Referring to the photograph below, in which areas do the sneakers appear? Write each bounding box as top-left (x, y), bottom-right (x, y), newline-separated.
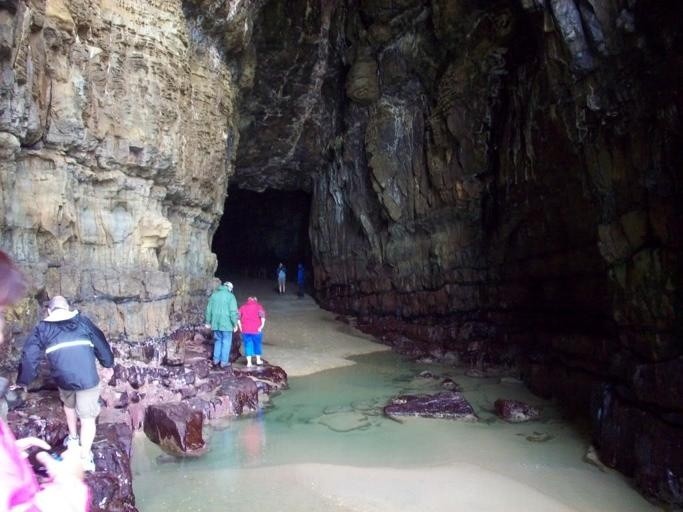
top-left (65, 437), bottom-right (78, 454)
top-left (247, 364), bottom-right (251, 368)
top-left (220, 361), bottom-right (231, 368)
top-left (80, 454), bottom-right (94, 472)
top-left (212, 360), bottom-right (219, 366)
top-left (257, 361), bottom-right (263, 365)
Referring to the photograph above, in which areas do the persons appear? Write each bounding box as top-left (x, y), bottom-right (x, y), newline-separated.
top-left (236, 295), bottom-right (266, 369)
top-left (7, 294), bottom-right (114, 456)
top-left (295, 262), bottom-right (305, 298)
top-left (275, 261), bottom-right (288, 297)
top-left (0, 251), bottom-right (96, 511)
top-left (238, 263), bottom-right (267, 281)
top-left (203, 281), bottom-right (238, 371)
